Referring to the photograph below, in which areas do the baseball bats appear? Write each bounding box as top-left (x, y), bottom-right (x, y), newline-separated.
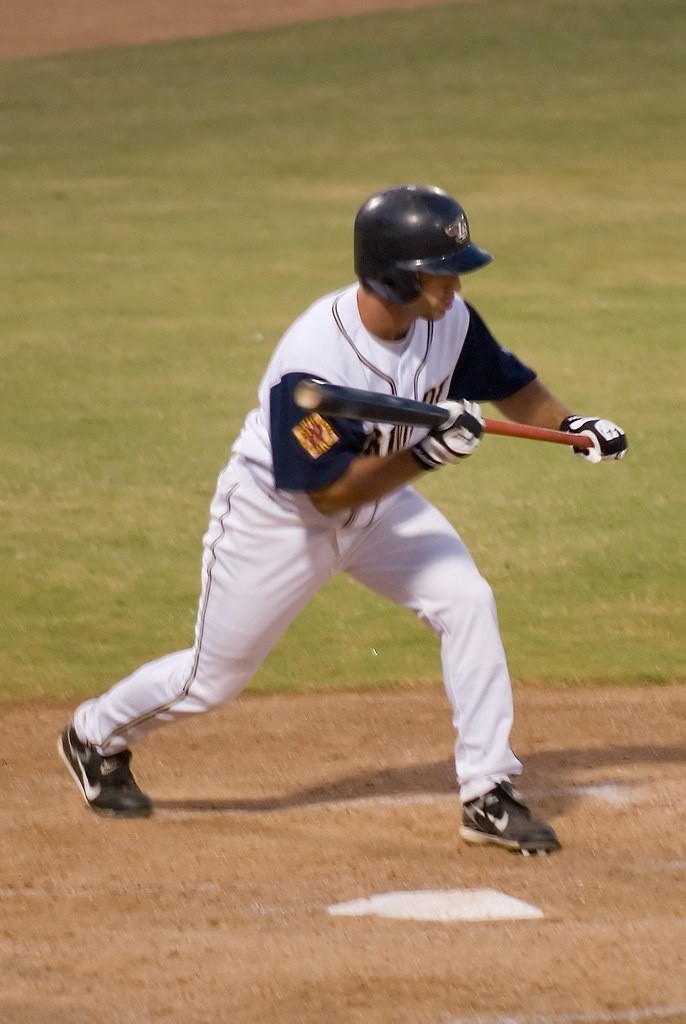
top-left (293, 375), bottom-right (593, 450)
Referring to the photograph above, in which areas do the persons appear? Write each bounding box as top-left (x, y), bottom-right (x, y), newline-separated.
top-left (58, 184), bottom-right (632, 854)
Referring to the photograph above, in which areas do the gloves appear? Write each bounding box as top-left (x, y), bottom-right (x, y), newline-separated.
top-left (559, 415), bottom-right (628, 463)
top-left (413, 398), bottom-right (488, 472)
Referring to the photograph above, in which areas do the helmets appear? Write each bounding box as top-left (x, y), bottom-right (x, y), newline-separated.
top-left (353, 186), bottom-right (491, 304)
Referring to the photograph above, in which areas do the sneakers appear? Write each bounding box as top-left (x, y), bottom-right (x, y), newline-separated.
top-left (461, 781), bottom-right (560, 856)
top-left (56, 720), bottom-right (154, 817)
